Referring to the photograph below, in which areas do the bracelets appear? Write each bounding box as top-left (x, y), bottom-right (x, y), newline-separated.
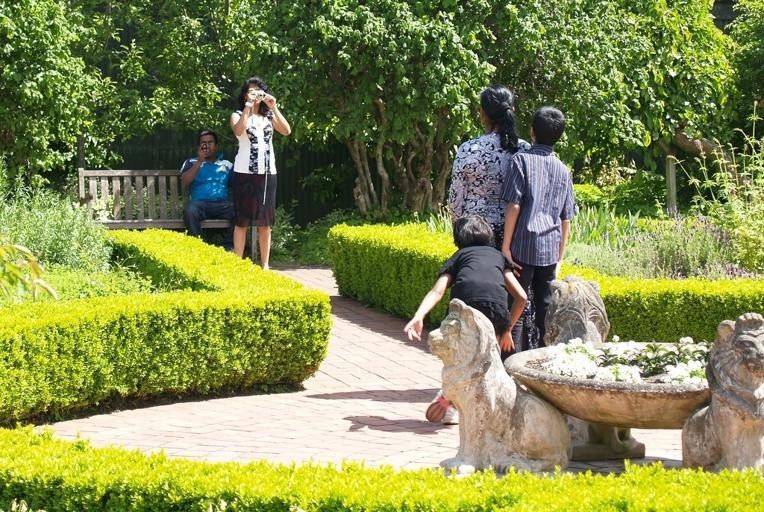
top-left (244, 100), bottom-right (254, 107)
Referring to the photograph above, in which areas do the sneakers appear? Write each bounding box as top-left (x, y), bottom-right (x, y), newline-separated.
top-left (426, 390), bottom-right (459, 425)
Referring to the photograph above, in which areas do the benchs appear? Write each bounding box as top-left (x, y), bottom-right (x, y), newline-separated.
top-left (76, 167), bottom-right (258, 265)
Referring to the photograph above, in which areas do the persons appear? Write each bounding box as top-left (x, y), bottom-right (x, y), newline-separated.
top-left (177, 129), bottom-right (239, 254)
top-left (448, 80), bottom-right (534, 262)
top-left (229, 76), bottom-right (291, 270)
top-left (502, 105), bottom-right (576, 356)
top-left (402, 212), bottom-right (529, 426)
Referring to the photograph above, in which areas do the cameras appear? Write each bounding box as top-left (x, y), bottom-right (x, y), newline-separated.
top-left (253, 89), bottom-right (267, 101)
